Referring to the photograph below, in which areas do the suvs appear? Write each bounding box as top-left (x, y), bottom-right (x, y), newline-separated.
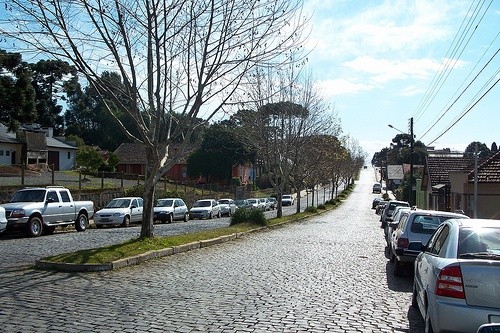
top-left (388, 210), bottom-right (471, 277)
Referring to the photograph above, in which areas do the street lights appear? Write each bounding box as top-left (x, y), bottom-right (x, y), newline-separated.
top-left (387, 124), bottom-right (414, 207)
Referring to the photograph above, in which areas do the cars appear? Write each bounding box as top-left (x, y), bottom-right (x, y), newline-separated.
top-left (407, 218), bottom-right (500, 333)
top-left (282, 195), bottom-right (294, 206)
top-left (234, 198), bottom-right (279, 212)
top-left (153, 198), bottom-right (190, 224)
top-left (217, 199), bottom-right (238, 217)
top-left (189, 199), bottom-right (222, 220)
top-left (93, 197), bottom-right (144, 229)
top-left (372, 197), bottom-right (441, 256)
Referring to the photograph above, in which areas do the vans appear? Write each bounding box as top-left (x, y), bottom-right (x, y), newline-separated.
top-left (373, 183), bottom-right (381, 193)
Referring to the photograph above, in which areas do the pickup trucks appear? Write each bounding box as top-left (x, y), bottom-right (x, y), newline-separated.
top-left (0, 186), bottom-right (95, 238)
